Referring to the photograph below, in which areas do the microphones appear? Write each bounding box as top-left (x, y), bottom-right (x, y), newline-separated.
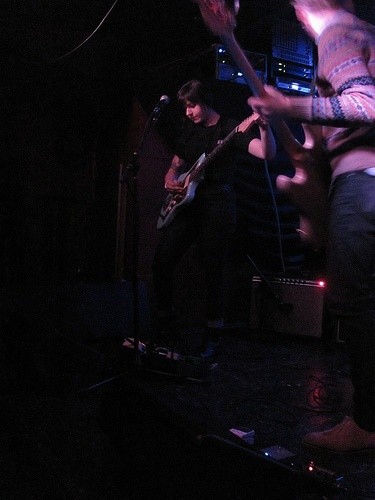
top-left (152, 95), bottom-right (170, 120)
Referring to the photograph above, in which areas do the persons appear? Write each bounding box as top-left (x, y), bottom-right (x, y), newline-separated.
top-left (247, 0), bottom-right (375, 447)
top-left (143, 80), bottom-right (276, 351)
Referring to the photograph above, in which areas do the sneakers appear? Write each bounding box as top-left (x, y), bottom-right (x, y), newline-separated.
top-left (307, 367), bottom-right (329, 411)
top-left (304, 416), bottom-right (375, 452)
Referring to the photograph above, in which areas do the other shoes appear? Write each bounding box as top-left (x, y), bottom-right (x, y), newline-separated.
top-left (207, 330), bottom-right (222, 356)
top-left (149, 332), bottom-right (162, 348)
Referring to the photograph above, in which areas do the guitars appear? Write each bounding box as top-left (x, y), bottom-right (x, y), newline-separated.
top-left (155, 112), bottom-right (258, 233)
top-left (197, 0), bottom-right (331, 252)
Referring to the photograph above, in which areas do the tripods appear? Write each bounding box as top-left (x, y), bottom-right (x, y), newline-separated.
top-left (76, 115), bottom-right (212, 395)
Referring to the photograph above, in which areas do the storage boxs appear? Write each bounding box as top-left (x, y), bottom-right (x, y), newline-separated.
top-left (249, 276), bottom-right (324, 338)
